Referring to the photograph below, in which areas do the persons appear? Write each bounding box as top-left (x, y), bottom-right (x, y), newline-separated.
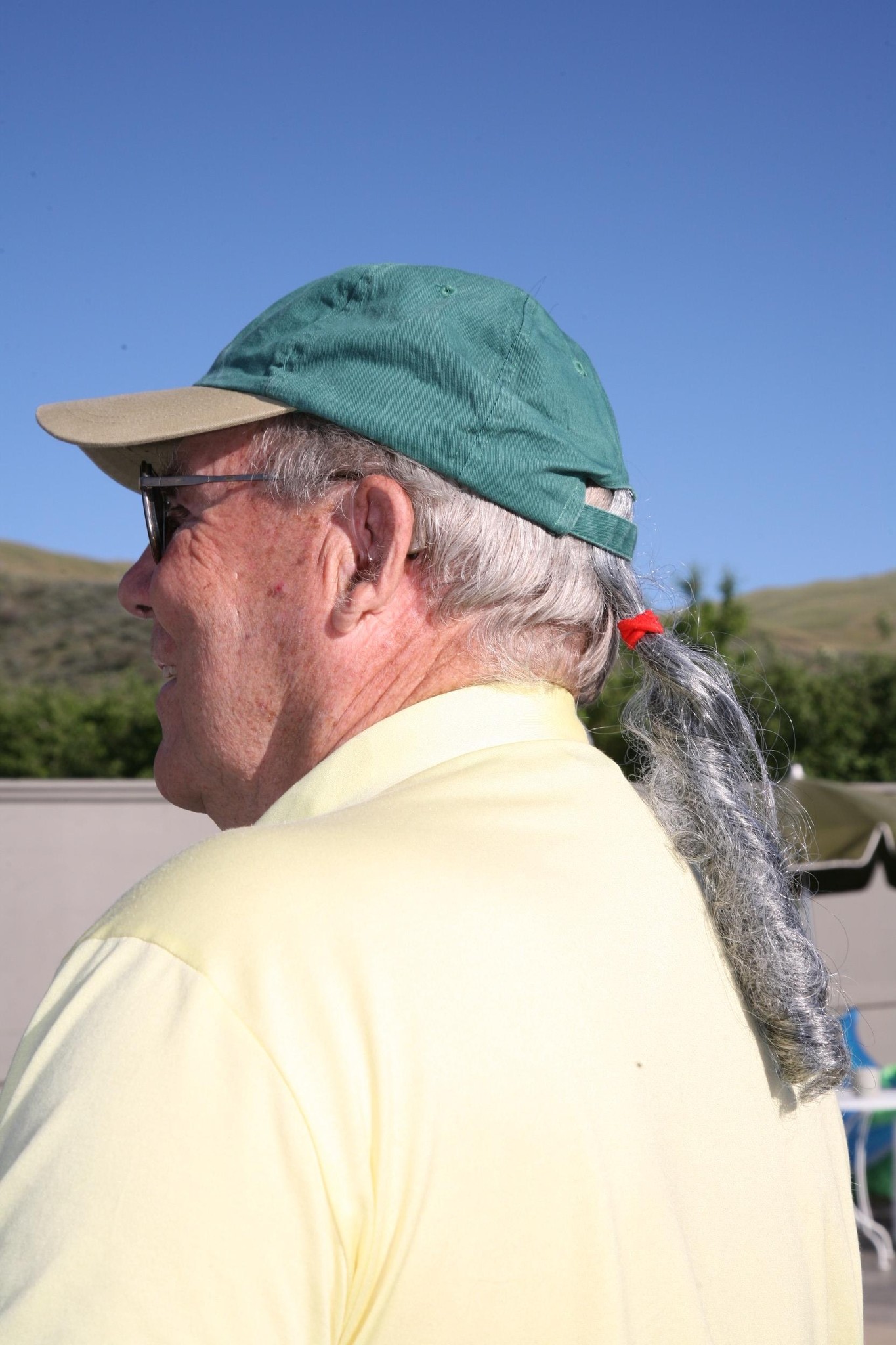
top-left (0, 268), bottom-right (869, 1344)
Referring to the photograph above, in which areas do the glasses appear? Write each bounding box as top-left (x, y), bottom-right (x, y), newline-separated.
top-left (136, 461), bottom-right (379, 552)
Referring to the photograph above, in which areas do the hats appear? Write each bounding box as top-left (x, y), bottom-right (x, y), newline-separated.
top-left (41, 266), bottom-right (646, 559)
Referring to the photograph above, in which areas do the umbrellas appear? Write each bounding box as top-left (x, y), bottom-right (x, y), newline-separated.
top-left (755, 758), bottom-right (896, 939)
